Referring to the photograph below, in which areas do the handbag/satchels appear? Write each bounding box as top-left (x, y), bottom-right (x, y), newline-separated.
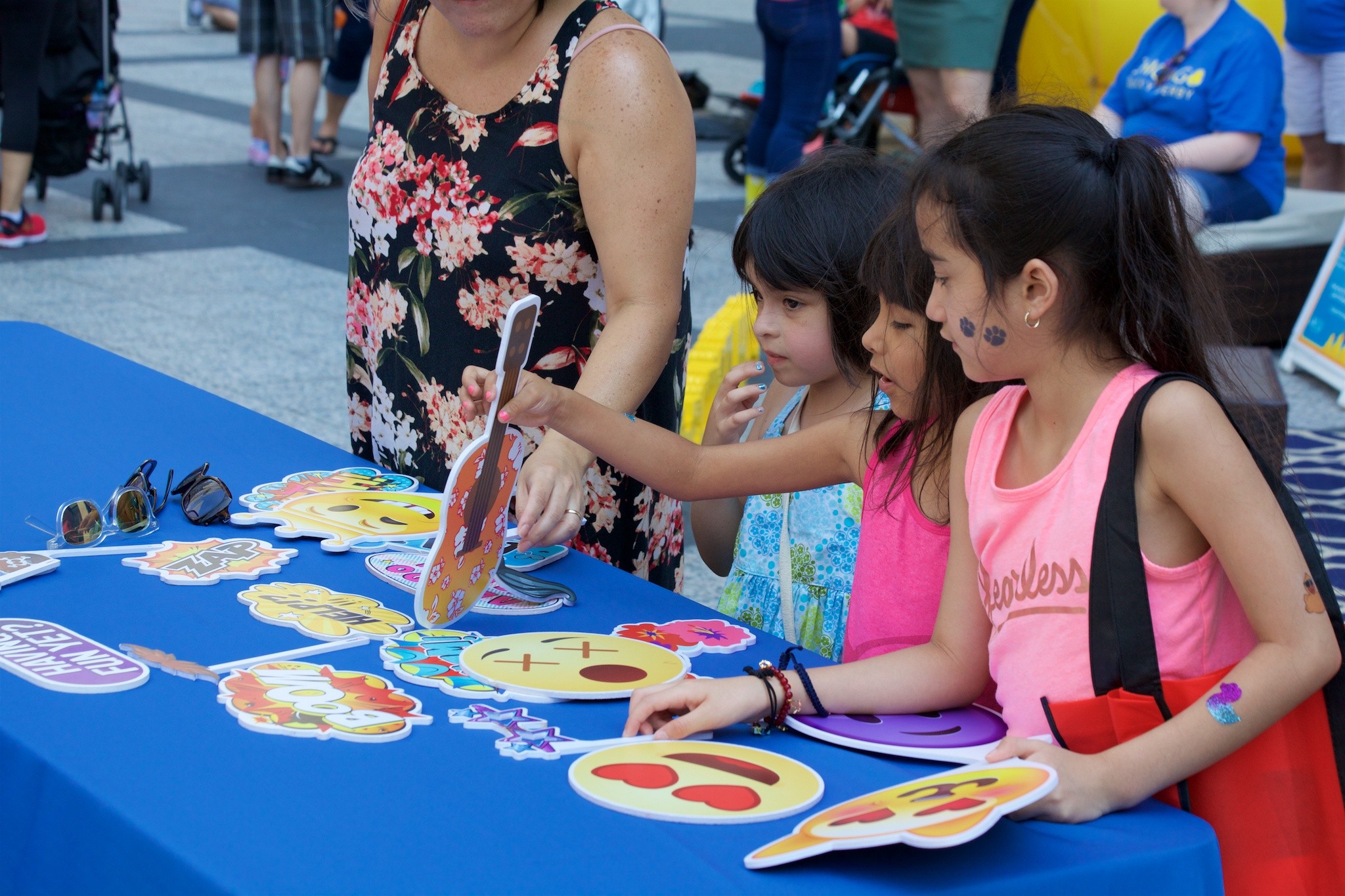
top-left (1039, 375), bottom-right (1345, 896)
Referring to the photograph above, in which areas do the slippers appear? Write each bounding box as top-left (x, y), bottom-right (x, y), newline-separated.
top-left (311, 135), bottom-right (339, 155)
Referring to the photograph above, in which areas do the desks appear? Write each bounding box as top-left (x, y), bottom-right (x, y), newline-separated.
top-left (0, 321), bottom-right (1225, 896)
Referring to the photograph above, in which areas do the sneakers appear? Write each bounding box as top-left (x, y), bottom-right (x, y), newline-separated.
top-left (1, 205), bottom-right (47, 247)
top-left (266, 139), bottom-right (290, 183)
top-left (282, 156), bottom-right (344, 188)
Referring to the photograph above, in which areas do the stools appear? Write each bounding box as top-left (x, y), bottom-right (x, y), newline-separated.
top-left (1194, 189), bottom-right (1345, 346)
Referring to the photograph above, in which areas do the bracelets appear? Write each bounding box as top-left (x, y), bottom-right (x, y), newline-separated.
top-left (740, 645), bottom-right (831, 737)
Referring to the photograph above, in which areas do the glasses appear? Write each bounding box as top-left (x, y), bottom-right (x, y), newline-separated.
top-left (25, 485), bottom-right (160, 550)
top-left (124, 459), bottom-right (174, 518)
top-left (171, 463), bottom-right (233, 526)
top-left (1158, 52), bottom-right (1186, 86)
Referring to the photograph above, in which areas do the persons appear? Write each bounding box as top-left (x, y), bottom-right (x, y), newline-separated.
top-left (183, 0), bottom-right (373, 190)
top-left (686, 147), bottom-right (917, 659)
top-left (350, 0), bottom-right (686, 588)
top-left (459, 185), bottom-right (992, 711)
top-left (725, 0), bottom-right (1345, 243)
top-left (619, 102), bottom-right (1334, 895)
top-left (1, 2), bottom-right (47, 251)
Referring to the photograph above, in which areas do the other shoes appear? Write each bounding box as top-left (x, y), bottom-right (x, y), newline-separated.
top-left (248, 140), bottom-right (269, 169)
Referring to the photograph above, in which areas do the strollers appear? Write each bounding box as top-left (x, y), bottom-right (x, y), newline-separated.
top-left (718, 0), bottom-right (910, 188)
top-left (21, 0), bottom-right (153, 222)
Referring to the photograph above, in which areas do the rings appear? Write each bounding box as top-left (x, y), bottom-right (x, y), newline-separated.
top-left (563, 508), bottom-right (583, 520)
